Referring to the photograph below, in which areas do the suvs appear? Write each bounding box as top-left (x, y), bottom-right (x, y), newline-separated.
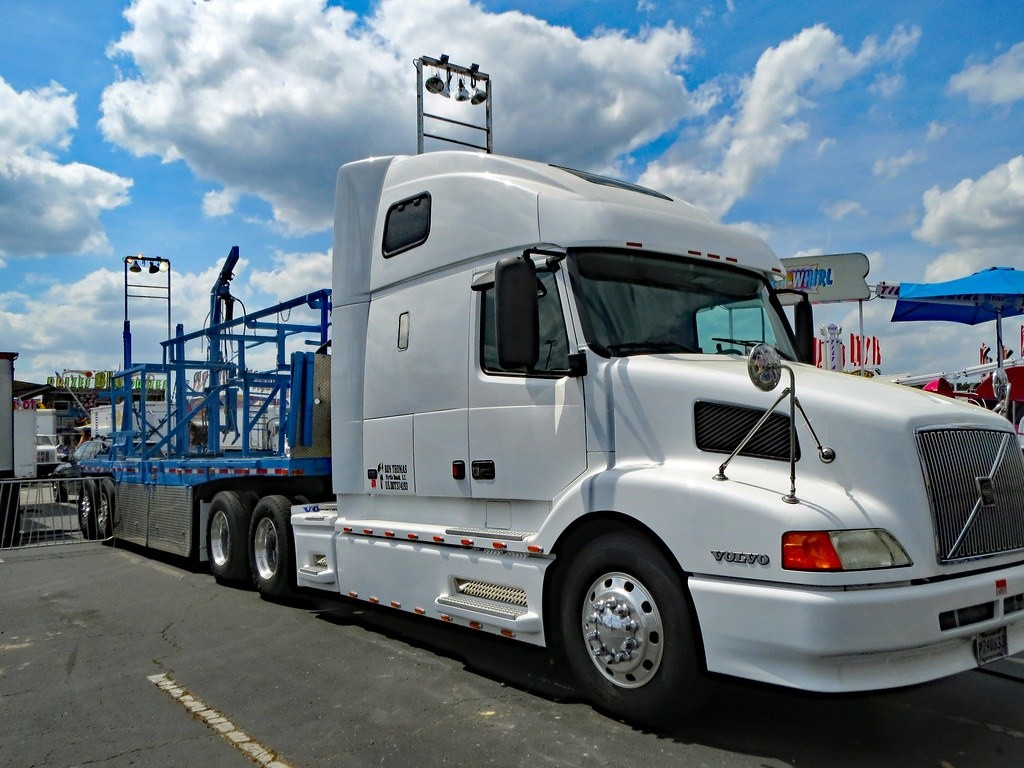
top-left (49, 440), bottom-right (169, 503)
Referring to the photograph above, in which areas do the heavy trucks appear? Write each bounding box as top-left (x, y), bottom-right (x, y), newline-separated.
top-left (72, 152), bottom-right (1023, 727)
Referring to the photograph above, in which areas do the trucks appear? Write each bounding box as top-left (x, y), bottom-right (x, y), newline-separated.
top-left (89, 400), bottom-right (178, 452)
top-left (35, 408), bottom-right (64, 466)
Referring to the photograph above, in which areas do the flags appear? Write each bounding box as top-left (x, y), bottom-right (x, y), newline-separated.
top-left (1020, 325), bottom-right (1024, 357)
top-left (813, 333), bottom-right (881, 368)
top-left (1003, 345), bottom-right (1014, 359)
top-left (980, 343), bottom-right (993, 365)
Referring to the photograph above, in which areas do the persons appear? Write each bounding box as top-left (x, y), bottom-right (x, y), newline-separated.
top-left (77, 428), bottom-right (92, 447)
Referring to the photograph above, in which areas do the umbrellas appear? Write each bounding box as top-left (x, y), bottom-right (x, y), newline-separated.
top-left (891, 266), bottom-right (1024, 367)
